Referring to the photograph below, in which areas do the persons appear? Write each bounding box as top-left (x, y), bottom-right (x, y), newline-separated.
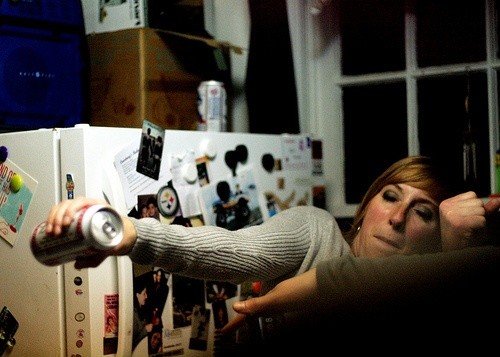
top-left (213, 191), bottom-right (500, 356)
top-left (106, 181), bottom-right (252, 357)
top-left (45, 156), bottom-right (447, 298)
top-left (139, 125), bottom-right (163, 173)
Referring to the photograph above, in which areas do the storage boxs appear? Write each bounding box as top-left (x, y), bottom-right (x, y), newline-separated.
top-left (0, 0), bottom-right (248, 130)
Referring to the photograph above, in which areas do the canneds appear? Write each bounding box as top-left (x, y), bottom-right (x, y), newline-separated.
top-left (30, 205), bottom-right (125, 266)
top-left (190, 81), bottom-right (228, 133)
